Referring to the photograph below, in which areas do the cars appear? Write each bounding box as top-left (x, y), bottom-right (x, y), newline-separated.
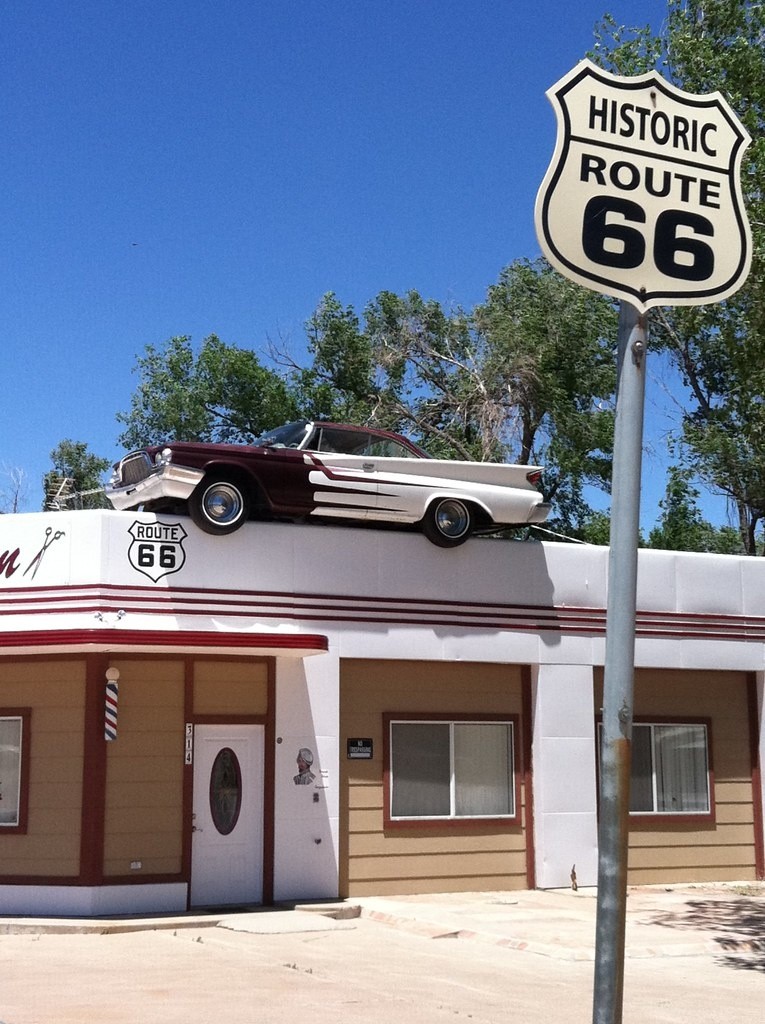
top-left (103, 420), bottom-right (559, 548)
